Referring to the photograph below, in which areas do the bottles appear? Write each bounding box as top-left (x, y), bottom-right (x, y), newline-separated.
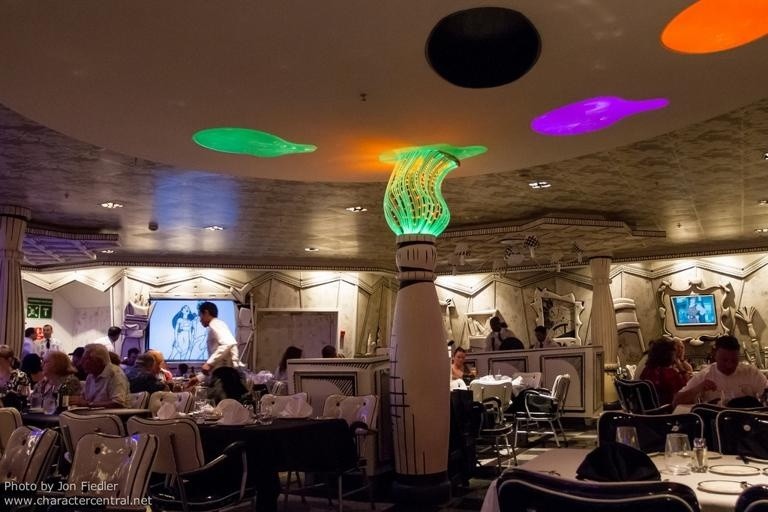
top-left (14, 383), bottom-right (81, 417)
top-left (693, 437), bottom-right (709, 474)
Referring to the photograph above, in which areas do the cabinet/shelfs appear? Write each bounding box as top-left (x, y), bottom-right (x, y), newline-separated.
top-left (466, 342), bottom-right (604, 430)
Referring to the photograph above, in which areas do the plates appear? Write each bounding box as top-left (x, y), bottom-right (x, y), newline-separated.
top-left (697, 479), bottom-right (752, 495)
top-left (646, 450), bottom-right (661, 459)
top-left (309, 415), bottom-right (335, 420)
top-left (762, 467), bottom-right (768, 476)
top-left (685, 449), bottom-right (721, 459)
top-left (707, 464), bottom-right (759, 476)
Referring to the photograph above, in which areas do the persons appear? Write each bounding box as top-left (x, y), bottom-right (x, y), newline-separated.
top-left (688, 299), bottom-right (706, 324)
top-left (0, 302), bottom-right (384, 427)
top-left (448, 313), bottom-right (768, 421)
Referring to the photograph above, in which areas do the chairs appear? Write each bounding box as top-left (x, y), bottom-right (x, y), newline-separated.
top-left (0, 357), bottom-right (768, 512)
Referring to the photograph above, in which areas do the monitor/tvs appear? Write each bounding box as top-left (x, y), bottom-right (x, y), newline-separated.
top-left (671, 293), bottom-right (718, 326)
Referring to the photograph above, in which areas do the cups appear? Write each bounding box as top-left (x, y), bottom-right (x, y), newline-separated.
top-left (495, 374), bottom-right (502, 381)
top-left (189, 387), bottom-right (275, 426)
top-left (663, 432), bottom-right (693, 475)
top-left (170, 369), bottom-right (201, 382)
top-left (613, 426), bottom-right (638, 451)
